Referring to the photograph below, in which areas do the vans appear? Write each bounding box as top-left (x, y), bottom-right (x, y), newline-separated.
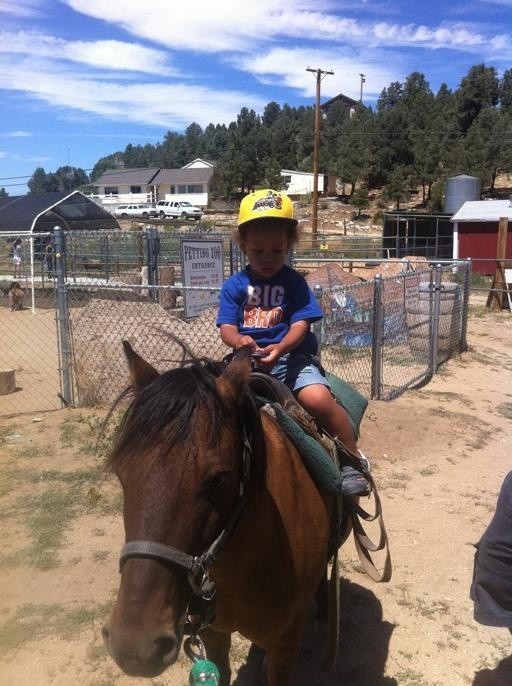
top-left (114, 204), bottom-right (157, 220)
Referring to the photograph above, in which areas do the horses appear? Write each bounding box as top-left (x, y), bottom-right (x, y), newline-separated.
top-left (79, 255), bottom-right (103, 272)
top-left (99, 328), bottom-right (360, 686)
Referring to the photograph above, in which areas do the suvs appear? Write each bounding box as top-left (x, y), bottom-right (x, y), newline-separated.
top-left (156, 200), bottom-right (202, 220)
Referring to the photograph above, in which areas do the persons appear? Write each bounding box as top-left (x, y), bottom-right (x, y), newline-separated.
top-left (13, 238), bottom-right (23, 279)
top-left (43, 237), bottom-right (55, 278)
top-left (216, 188), bottom-right (373, 497)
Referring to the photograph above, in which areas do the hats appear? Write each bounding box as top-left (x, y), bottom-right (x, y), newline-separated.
top-left (238, 189), bottom-right (299, 231)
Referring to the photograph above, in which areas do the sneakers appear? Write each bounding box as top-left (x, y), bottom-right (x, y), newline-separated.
top-left (341, 458), bottom-right (371, 496)
